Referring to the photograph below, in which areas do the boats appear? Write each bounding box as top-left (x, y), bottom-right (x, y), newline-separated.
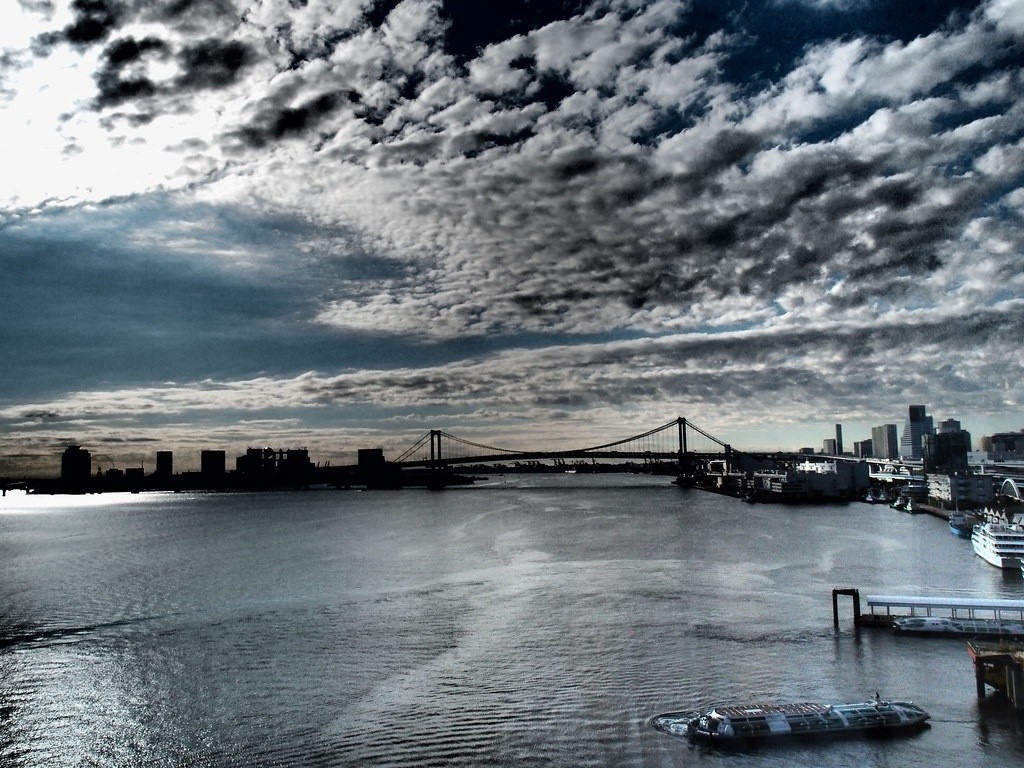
top-left (889, 497), bottom-right (905, 510)
top-left (946, 619), bottom-right (1009, 635)
top-left (971, 521), bottom-right (1024, 568)
top-left (889, 617), bottom-right (951, 634)
top-left (1005, 623), bottom-right (1024, 641)
top-left (949, 511), bottom-right (969, 537)
top-left (904, 497), bottom-right (920, 513)
top-left (687, 692), bottom-right (931, 746)
